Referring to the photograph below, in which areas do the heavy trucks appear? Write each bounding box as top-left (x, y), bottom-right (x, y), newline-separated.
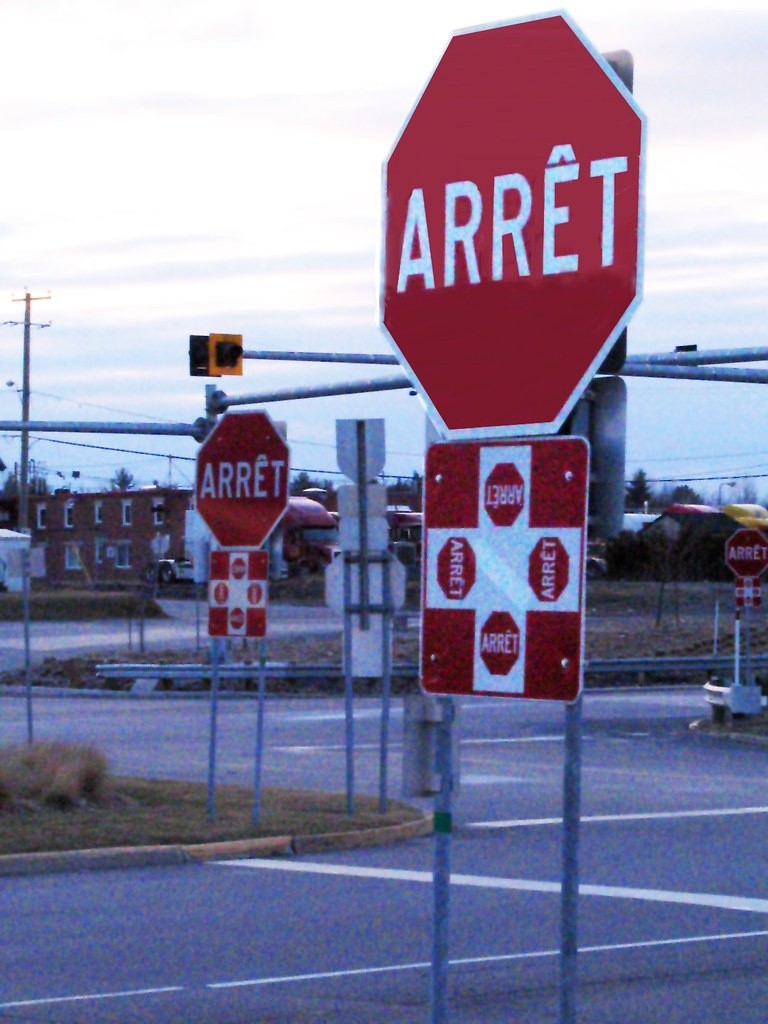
top-left (175, 496), bottom-right (422, 579)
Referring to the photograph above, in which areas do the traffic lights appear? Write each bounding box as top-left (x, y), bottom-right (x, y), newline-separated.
top-left (206, 333), bottom-right (244, 378)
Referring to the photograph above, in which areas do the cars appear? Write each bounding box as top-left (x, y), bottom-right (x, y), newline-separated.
top-left (143, 558), bottom-right (289, 584)
